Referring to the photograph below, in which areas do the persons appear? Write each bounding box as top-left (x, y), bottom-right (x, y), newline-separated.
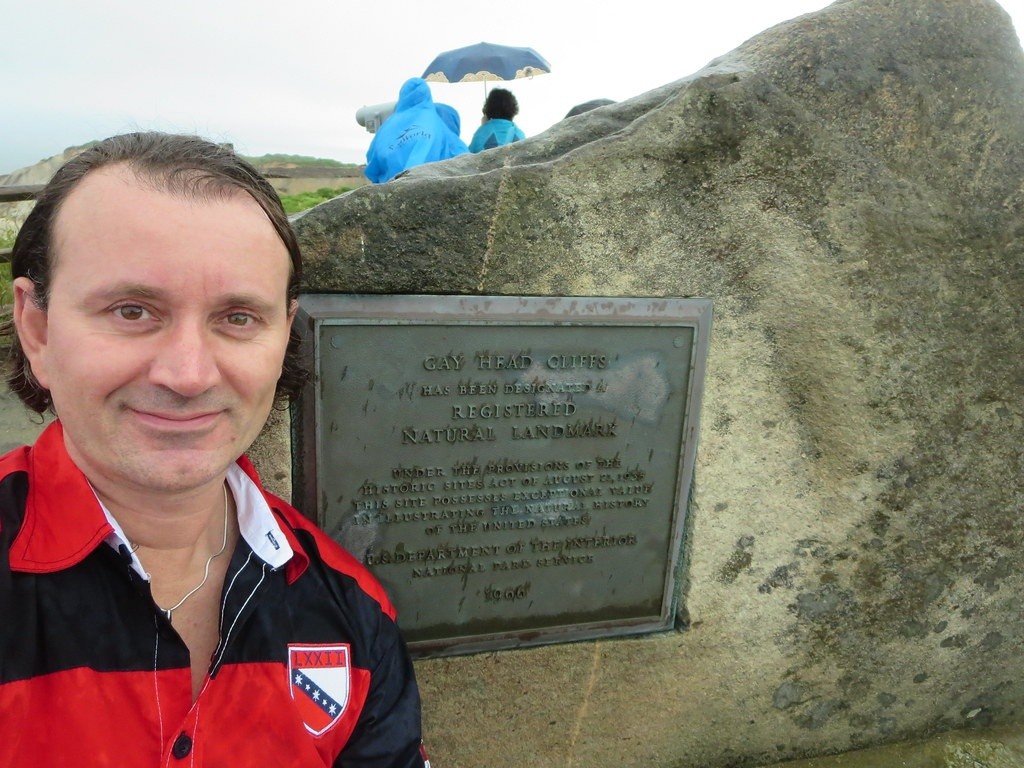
top-left (366, 77), bottom-right (469, 186)
top-left (468, 88), bottom-right (526, 154)
top-left (1, 130), bottom-right (424, 768)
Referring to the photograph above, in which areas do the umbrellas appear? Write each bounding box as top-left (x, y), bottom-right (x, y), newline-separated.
top-left (421, 40), bottom-right (552, 100)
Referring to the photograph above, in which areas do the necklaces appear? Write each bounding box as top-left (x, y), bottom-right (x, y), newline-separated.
top-left (157, 483), bottom-right (227, 621)
